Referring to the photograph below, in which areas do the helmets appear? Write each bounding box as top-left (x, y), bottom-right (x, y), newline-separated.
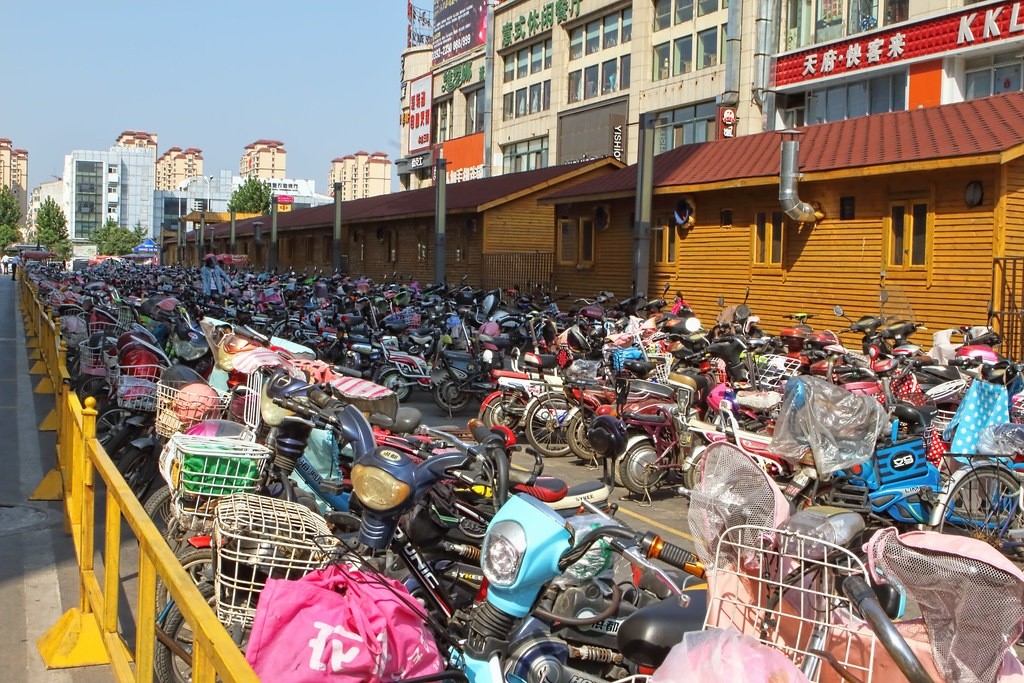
top-left (88, 331), bottom-right (105, 354)
top-left (182, 418), bottom-right (246, 437)
top-left (108, 345), bottom-right (118, 356)
top-left (479, 321), bottom-right (500, 338)
top-left (160, 364), bottom-right (222, 425)
top-left (117, 340), bottom-right (161, 384)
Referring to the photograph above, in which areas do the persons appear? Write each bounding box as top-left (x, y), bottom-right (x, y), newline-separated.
top-left (201, 253), bottom-right (234, 305)
top-left (0, 253), bottom-right (21, 281)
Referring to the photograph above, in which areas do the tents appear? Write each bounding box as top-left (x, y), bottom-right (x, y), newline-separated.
top-left (130, 238), bottom-right (158, 262)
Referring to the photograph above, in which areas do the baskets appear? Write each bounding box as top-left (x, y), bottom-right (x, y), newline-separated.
top-left (312, 534), bottom-right (466, 672)
top-left (211, 491), bottom-right (343, 630)
top-left (78, 322), bottom-right (118, 377)
top-left (753, 353), bottom-right (803, 396)
top-left (65, 309), bottom-right (89, 336)
top-left (114, 333), bottom-right (173, 412)
top-left (155, 376), bottom-right (232, 439)
top-left (696, 523), bottom-right (877, 683)
top-left (102, 336), bottom-right (120, 386)
top-left (166, 432), bottom-right (274, 533)
top-left (645, 351), bottom-right (674, 381)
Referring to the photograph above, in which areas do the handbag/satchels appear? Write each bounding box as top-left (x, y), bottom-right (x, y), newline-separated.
top-left (244, 559), bottom-right (445, 682)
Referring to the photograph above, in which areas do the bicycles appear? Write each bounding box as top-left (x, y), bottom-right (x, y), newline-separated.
top-left (24, 254), bottom-right (1021, 682)
